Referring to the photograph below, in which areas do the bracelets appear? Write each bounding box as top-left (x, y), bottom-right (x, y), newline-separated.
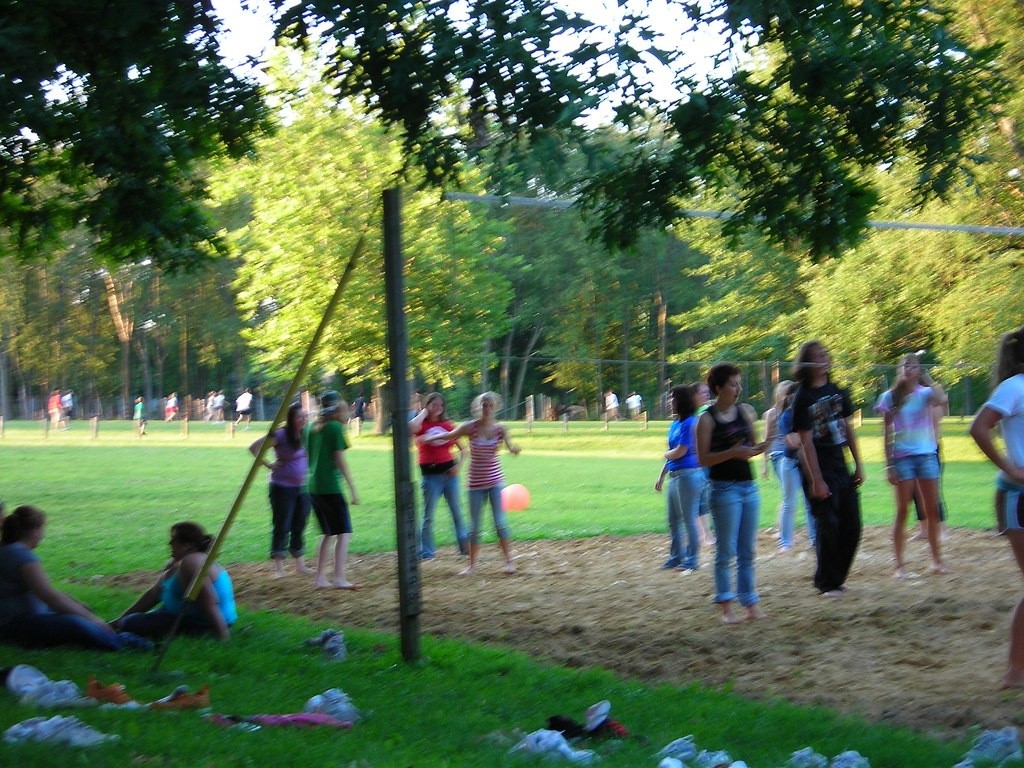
top-left (930, 382), bottom-right (938, 388)
top-left (885, 464), bottom-right (896, 469)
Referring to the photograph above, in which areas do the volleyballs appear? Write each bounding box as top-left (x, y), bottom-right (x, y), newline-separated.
top-left (502, 484), bottom-right (531, 511)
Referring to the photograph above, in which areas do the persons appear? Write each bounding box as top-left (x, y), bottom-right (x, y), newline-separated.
top-left (348, 393), bottom-right (367, 428)
top-left (626, 391), bottom-right (645, 420)
top-left (693, 381), bottom-right (716, 544)
top-left (970, 328), bottom-right (1024, 689)
top-left (49, 389), bottom-right (75, 431)
top-left (424, 392), bottom-right (524, 575)
top-left (904, 402), bottom-right (949, 551)
top-left (654, 384), bottom-right (708, 572)
top-left (0, 504), bottom-right (152, 652)
top-left (249, 402), bottom-right (313, 580)
top-left (874, 355), bottom-right (951, 580)
top-left (303, 389), bottom-right (365, 590)
top-left (409, 391), bottom-right (468, 558)
top-left (762, 379), bottom-right (794, 535)
top-left (133, 396), bottom-right (148, 435)
top-left (761, 340), bottom-right (867, 598)
top-left (776, 383), bottom-right (817, 554)
top-left (167, 387), bottom-right (253, 429)
top-left (107, 522), bottom-right (239, 642)
top-left (604, 391), bottom-right (620, 421)
top-left (696, 364), bottom-right (771, 625)
